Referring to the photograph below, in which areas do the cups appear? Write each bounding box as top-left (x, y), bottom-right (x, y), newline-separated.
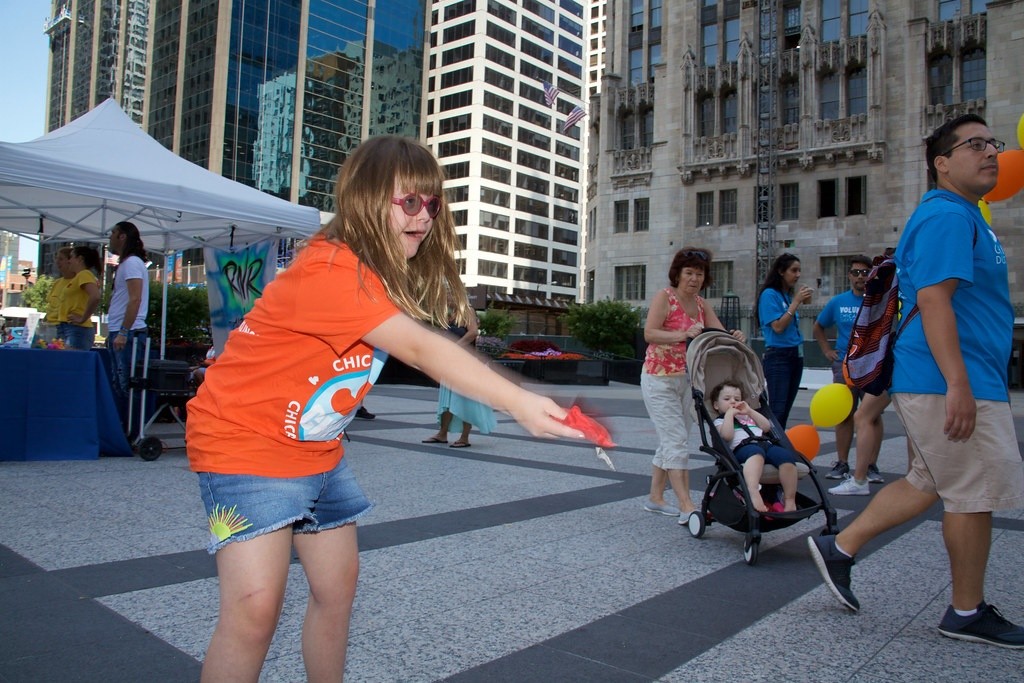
top-left (802, 289), bottom-right (812, 305)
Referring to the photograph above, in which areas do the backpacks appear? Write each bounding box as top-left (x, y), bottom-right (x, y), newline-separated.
top-left (846, 195), bottom-right (978, 397)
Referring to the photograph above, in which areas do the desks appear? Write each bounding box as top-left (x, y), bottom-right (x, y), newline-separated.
top-left (0, 346), bottom-right (136, 462)
top-left (140, 349), bottom-right (187, 421)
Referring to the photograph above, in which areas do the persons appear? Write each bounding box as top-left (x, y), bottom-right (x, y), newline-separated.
top-left (108, 222), bottom-right (150, 443)
top-left (808, 112), bottom-right (1024, 649)
top-left (184, 135), bottom-right (587, 682)
top-left (640, 246), bottom-right (747, 525)
top-left (45, 246), bottom-right (102, 350)
top-left (711, 377), bottom-right (799, 514)
top-left (757, 253), bottom-right (813, 433)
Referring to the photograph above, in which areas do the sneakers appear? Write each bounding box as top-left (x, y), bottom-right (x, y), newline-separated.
top-left (866, 463), bottom-right (884, 483)
top-left (643, 499), bottom-right (681, 517)
top-left (679, 509), bottom-right (702, 524)
top-left (354, 406), bottom-right (375, 419)
top-left (938, 604), bottom-right (1024, 649)
top-left (807, 535), bottom-right (861, 613)
top-left (826, 460), bottom-right (849, 479)
top-left (828, 476), bottom-right (870, 494)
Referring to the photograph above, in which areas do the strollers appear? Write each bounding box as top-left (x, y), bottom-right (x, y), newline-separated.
top-left (684, 326), bottom-right (841, 566)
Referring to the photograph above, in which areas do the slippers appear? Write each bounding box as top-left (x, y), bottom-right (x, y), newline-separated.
top-left (450, 441), bottom-right (471, 448)
top-left (422, 437), bottom-right (448, 443)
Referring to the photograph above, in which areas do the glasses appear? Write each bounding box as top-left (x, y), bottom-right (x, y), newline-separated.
top-left (941, 137), bottom-right (1005, 156)
top-left (784, 253), bottom-right (792, 257)
top-left (849, 269), bottom-right (871, 277)
top-left (682, 251), bottom-right (709, 260)
top-left (391, 193), bottom-right (441, 218)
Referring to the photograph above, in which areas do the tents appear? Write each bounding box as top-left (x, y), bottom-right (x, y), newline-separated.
top-left (0, 95), bottom-right (319, 364)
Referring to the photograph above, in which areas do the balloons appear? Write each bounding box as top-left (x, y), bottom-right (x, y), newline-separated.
top-left (785, 421), bottom-right (822, 463)
top-left (977, 196), bottom-right (992, 228)
top-left (1016, 112), bottom-right (1024, 150)
top-left (809, 383), bottom-right (854, 429)
top-left (981, 149), bottom-right (1024, 202)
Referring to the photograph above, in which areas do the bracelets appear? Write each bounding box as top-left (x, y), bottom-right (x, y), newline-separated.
top-left (119, 326), bottom-right (130, 333)
top-left (787, 309), bottom-right (793, 319)
top-left (119, 332), bottom-right (127, 336)
